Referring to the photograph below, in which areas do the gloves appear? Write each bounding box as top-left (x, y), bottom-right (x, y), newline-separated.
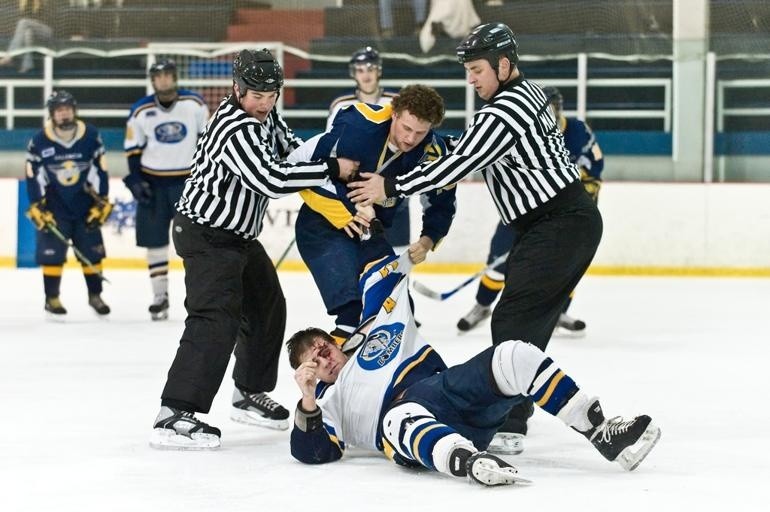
top-left (24, 201), bottom-right (52, 234)
top-left (129, 179), bottom-right (155, 206)
top-left (86, 198), bottom-right (114, 228)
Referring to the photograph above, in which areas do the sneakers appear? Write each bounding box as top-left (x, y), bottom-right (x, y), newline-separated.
top-left (555, 312), bottom-right (589, 331)
top-left (148, 294), bottom-right (170, 314)
top-left (88, 296), bottom-right (107, 311)
top-left (456, 303), bottom-right (490, 331)
top-left (229, 386), bottom-right (289, 422)
top-left (154, 407), bottom-right (221, 443)
top-left (46, 300), bottom-right (64, 315)
top-left (449, 447), bottom-right (511, 482)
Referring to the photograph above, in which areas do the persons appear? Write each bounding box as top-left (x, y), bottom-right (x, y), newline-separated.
top-left (285, 203), bottom-right (652, 488)
top-left (0, 0), bottom-right (62, 75)
top-left (123, 60), bottom-right (211, 314)
top-left (456, 86), bottom-right (604, 330)
top-left (25, 91), bottom-right (113, 314)
top-left (154, 48), bottom-right (360, 439)
top-left (346, 22), bottom-right (603, 435)
top-left (325, 46), bottom-right (401, 134)
top-left (377, 0), bottom-right (426, 38)
top-left (287, 84), bottom-right (457, 351)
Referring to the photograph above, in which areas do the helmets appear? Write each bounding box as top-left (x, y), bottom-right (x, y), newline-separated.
top-left (147, 57), bottom-right (181, 93)
top-left (47, 89), bottom-right (77, 130)
top-left (230, 47), bottom-right (286, 105)
top-left (455, 19), bottom-right (524, 87)
top-left (346, 45), bottom-right (384, 95)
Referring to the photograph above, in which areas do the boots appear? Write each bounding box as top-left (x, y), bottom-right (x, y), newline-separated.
top-left (571, 399), bottom-right (651, 465)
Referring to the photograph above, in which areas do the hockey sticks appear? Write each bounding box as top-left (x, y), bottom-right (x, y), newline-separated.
top-left (413, 252), bottom-right (509, 301)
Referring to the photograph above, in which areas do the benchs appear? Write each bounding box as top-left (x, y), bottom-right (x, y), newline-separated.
top-left (1, 0), bottom-right (239, 130)
top-left (283, 1), bottom-right (770, 130)
